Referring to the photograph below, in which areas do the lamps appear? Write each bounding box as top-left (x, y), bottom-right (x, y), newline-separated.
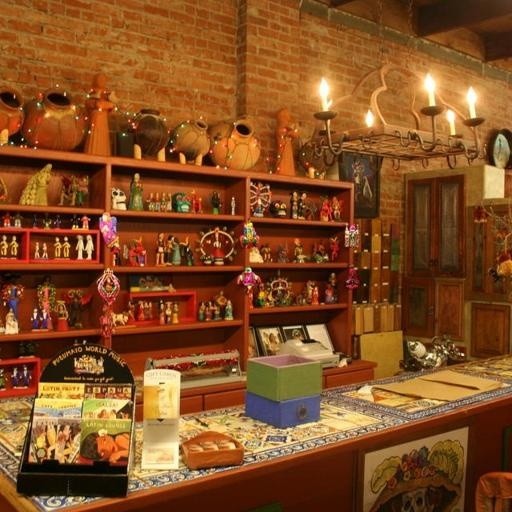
top-left (306, 0), bottom-right (492, 172)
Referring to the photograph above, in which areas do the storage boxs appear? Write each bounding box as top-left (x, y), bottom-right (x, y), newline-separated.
top-left (245, 350), bottom-right (322, 404)
top-left (243, 388), bottom-right (321, 431)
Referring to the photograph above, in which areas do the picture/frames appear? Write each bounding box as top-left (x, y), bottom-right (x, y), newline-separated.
top-left (277, 322), bottom-right (307, 344)
top-left (256, 325), bottom-right (285, 357)
top-left (246, 325), bottom-right (260, 358)
top-left (304, 321), bottom-right (337, 354)
top-left (352, 413), bottom-right (480, 512)
top-left (336, 147), bottom-right (380, 219)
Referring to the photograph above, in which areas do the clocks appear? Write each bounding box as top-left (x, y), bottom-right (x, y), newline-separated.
top-left (489, 128), bottom-right (512, 170)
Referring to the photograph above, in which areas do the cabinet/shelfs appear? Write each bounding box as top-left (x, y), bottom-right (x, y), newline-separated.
top-left (0, 137), bottom-right (379, 425)
top-left (400, 163), bottom-right (512, 360)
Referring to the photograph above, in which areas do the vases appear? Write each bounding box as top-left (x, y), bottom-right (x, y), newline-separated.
top-left (0, 79), bottom-right (267, 172)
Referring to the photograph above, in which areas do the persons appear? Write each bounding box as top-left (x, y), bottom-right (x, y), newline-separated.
top-left (0, 162), bottom-right (343, 389)
top-left (1, 165), bottom-right (96, 388)
top-left (264, 329), bottom-right (305, 357)
top-left (249, 191), bottom-right (342, 309)
top-left (79, 431), bottom-right (131, 464)
top-left (112, 172), bottom-right (236, 325)
